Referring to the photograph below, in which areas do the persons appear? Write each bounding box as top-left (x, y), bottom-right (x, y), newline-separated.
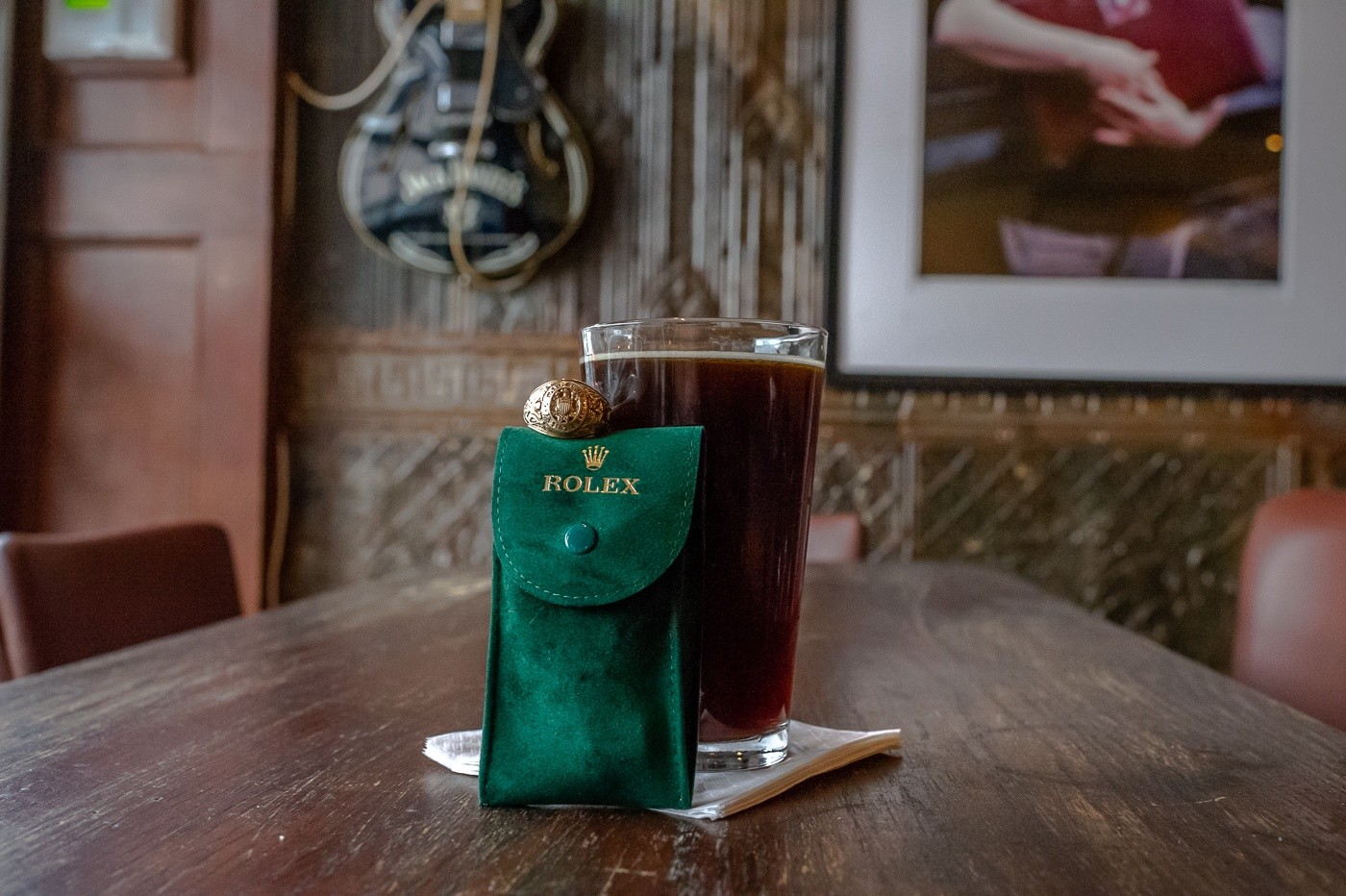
top-left (935, 0), bottom-right (1263, 278)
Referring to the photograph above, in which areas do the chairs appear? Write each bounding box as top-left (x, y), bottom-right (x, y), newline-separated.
top-left (0, 564), bottom-right (1346, 896)
top-left (1228, 489), bottom-right (1346, 731)
top-left (0, 520), bottom-right (249, 681)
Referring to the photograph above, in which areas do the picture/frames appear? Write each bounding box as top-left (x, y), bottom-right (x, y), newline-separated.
top-left (819, 0), bottom-right (1346, 401)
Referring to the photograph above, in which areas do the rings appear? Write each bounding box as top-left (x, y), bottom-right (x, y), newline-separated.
top-left (523, 378), bottom-right (612, 440)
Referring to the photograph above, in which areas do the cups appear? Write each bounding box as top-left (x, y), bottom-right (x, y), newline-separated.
top-left (577, 317), bottom-right (828, 773)
